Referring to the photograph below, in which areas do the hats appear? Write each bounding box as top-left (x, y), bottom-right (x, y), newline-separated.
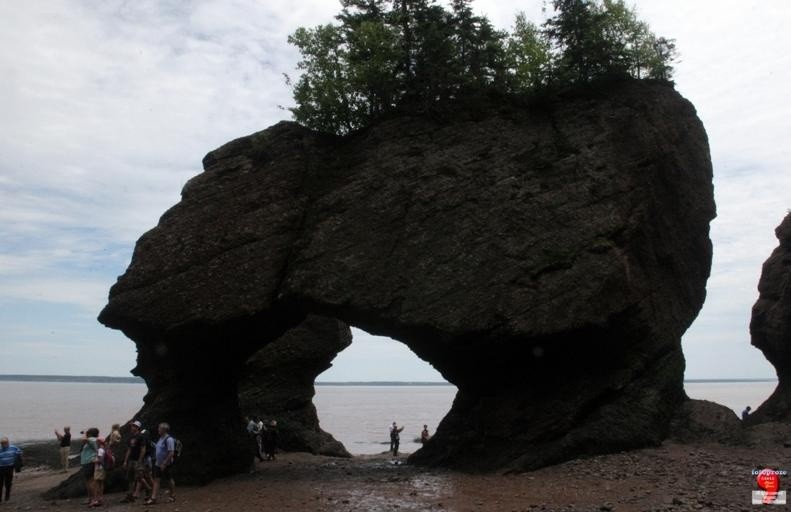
top-left (133, 420), bottom-right (141, 427)
top-left (140, 430), bottom-right (149, 436)
top-left (269, 420), bottom-right (277, 426)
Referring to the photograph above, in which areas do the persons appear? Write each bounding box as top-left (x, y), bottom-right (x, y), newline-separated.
top-left (0, 435), bottom-right (23, 504)
top-left (53, 425), bottom-right (73, 473)
top-left (247, 412), bottom-right (264, 458)
top-left (741, 404), bottom-right (753, 424)
top-left (79, 418), bottom-right (182, 505)
top-left (421, 423), bottom-right (430, 445)
top-left (389, 421), bottom-right (398, 451)
top-left (391, 425), bottom-right (406, 455)
top-left (261, 419), bottom-right (280, 461)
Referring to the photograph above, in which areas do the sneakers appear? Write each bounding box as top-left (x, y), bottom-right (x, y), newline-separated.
top-left (120, 492), bottom-right (139, 503)
top-left (143, 497), bottom-right (155, 505)
top-left (166, 497), bottom-right (175, 502)
top-left (83, 499), bottom-right (102, 507)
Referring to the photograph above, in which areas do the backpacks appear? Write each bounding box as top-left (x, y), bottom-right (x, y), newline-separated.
top-left (164, 436), bottom-right (182, 456)
top-left (102, 450), bottom-right (116, 470)
